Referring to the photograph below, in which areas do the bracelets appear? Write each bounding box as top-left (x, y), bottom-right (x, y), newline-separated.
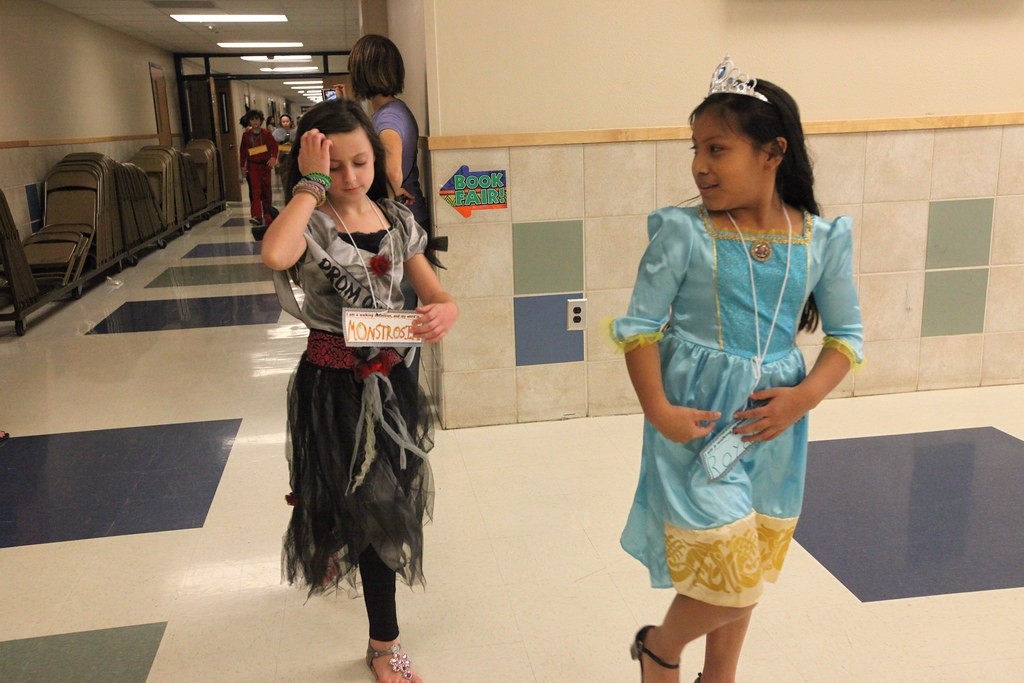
top-left (291, 172), bottom-right (333, 207)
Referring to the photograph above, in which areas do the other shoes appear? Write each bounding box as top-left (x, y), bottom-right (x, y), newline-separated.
top-left (250, 217), bottom-right (262, 225)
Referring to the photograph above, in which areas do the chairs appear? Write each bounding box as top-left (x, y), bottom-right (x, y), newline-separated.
top-left (0, 138), bottom-right (221, 311)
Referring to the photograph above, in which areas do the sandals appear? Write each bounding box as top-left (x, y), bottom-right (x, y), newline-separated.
top-left (632, 626), bottom-right (680, 683)
top-left (366, 639), bottom-right (412, 683)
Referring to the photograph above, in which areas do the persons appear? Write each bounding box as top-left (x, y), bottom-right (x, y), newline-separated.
top-left (238, 110), bottom-right (303, 222)
top-left (602, 54), bottom-right (865, 683)
top-left (259, 97), bottom-right (459, 683)
top-left (334, 34), bottom-right (433, 311)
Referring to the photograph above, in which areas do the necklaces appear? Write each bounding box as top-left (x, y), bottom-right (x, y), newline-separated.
top-left (734, 203), bottom-right (778, 262)
